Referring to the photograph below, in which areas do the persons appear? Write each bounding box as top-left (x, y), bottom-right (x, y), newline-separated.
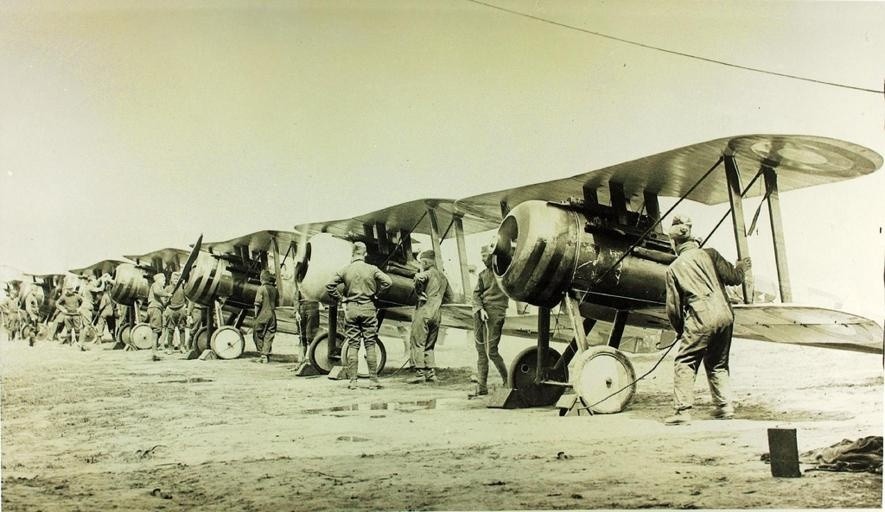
top-left (672, 214), bottom-right (703, 245)
top-left (467, 246), bottom-right (508, 397)
top-left (0, 270), bottom-right (213, 355)
top-left (406, 250), bottom-right (453, 383)
top-left (289, 289), bottom-right (320, 371)
top-left (252, 270), bottom-right (280, 363)
top-left (325, 242), bottom-right (392, 390)
top-left (663, 223), bottom-right (752, 424)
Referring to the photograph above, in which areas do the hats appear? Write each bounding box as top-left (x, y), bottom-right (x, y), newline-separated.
top-left (419, 250), bottom-right (434, 260)
top-left (353, 241), bottom-right (367, 255)
top-left (668, 224), bottom-right (691, 240)
top-left (481, 245), bottom-right (492, 254)
top-left (260, 269), bottom-right (270, 281)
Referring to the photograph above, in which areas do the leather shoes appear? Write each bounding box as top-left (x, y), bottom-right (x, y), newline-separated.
top-left (710, 405), bottom-right (734, 418)
top-left (153, 346), bottom-right (187, 361)
top-left (407, 375), bottom-right (426, 384)
top-left (426, 375), bottom-right (437, 382)
top-left (663, 411), bottom-right (691, 426)
top-left (348, 380), bottom-right (360, 390)
top-left (468, 389), bottom-right (488, 401)
top-left (251, 355), bottom-right (269, 364)
top-left (368, 378), bottom-right (383, 389)
